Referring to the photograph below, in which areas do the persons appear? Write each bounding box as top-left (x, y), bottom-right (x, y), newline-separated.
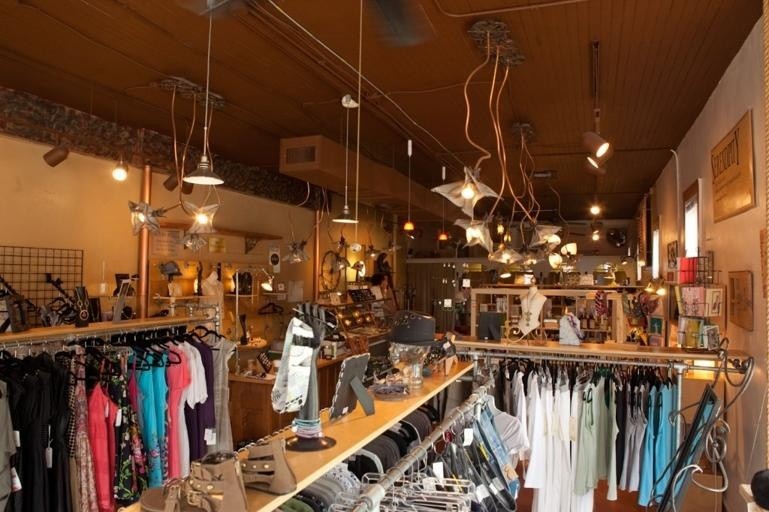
top-left (380, 274), bottom-right (396, 314)
top-left (202, 271), bottom-right (224, 341)
top-left (518, 285), bottom-right (548, 335)
top-left (365, 272), bottom-right (384, 319)
top-left (456, 271), bottom-right (471, 336)
top-left (381, 310), bottom-right (445, 385)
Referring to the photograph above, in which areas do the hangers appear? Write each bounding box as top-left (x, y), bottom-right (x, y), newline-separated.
top-left (0, 324), bottom-right (236, 390)
top-left (495, 351), bottom-right (680, 407)
top-left (294, 386), bottom-right (499, 512)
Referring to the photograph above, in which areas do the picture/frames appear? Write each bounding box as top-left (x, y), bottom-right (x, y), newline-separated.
top-left (710, 108), bottom-right (759, 331)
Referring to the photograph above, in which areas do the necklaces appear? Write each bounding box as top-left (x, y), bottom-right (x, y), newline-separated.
top-left (525, 292), bottom-right (539, 326)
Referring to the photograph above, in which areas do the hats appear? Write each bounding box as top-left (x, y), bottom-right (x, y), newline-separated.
top-left (382, 310), bottom-right (443, 347)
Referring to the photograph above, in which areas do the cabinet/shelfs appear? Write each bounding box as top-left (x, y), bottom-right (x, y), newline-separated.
top-left (472, 288), bottom-right (635, 344)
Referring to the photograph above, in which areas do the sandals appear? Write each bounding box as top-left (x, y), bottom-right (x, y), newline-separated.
top-left (140, 450), bottom-right (246, 512)
top-left (240, 439), bottom-right (297, 494)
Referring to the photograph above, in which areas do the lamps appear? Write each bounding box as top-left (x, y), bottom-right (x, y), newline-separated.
top-left (44, 144), bottom-right (70, 166)
top-left (253, 267), bottom-right (276, 294)
top-left (113, 3), bottom-right (221, 237)
